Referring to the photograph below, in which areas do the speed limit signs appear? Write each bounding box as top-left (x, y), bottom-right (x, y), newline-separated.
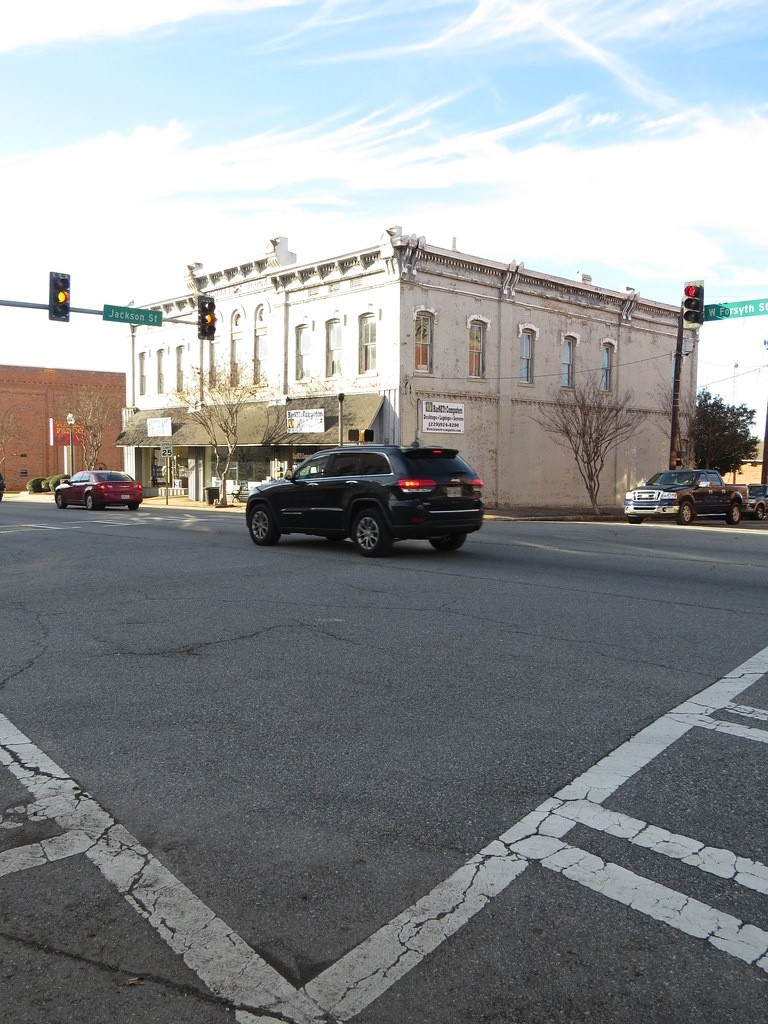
top-left (161, 440), bottom-right (174, 457)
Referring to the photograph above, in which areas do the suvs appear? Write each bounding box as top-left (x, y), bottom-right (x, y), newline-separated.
top-left (244, 443), bottom-right (486, 559)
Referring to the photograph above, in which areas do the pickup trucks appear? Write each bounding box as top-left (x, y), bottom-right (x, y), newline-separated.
top-left (624, 467), bottom-right (750, 525)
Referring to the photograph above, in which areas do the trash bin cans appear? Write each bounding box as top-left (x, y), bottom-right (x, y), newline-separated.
top-left (203, 487), bottom-right (220, 504)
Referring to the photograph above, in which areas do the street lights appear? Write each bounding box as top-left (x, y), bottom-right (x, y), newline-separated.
top-left (66, 413), bottom-right (75, 480)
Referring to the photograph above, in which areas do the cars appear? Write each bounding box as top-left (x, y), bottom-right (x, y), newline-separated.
top-left (54, 469), bottom-right (144, 511)
top-left (740, 484), bottom-right (768, 521)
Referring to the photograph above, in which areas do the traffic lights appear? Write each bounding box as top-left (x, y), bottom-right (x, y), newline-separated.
top-left (49, 271), bottom-right (71, 322)
top-left (197, 295), bottom-right (217, 342)
top-left (682, 279), bottom-right (704, 329)
